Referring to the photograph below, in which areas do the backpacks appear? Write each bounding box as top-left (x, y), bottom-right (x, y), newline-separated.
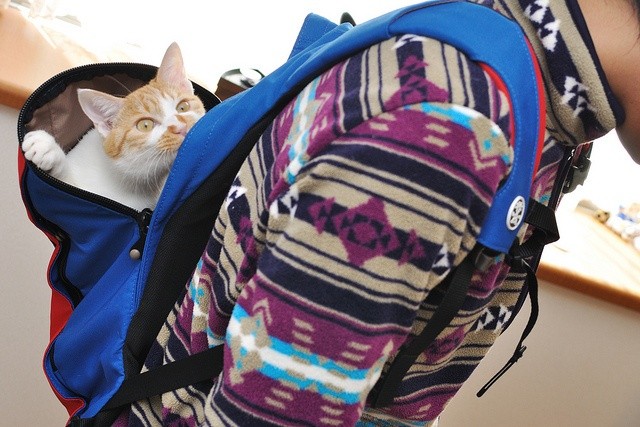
top-left (18, 0), bottom-right (560, 427)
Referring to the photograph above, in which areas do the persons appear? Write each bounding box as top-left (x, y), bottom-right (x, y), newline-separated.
top-left (116, 1), bottom-right (640, 427)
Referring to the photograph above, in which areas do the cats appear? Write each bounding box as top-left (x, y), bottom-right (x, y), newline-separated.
top-left (22, 41), bottom-right (206, 212)
